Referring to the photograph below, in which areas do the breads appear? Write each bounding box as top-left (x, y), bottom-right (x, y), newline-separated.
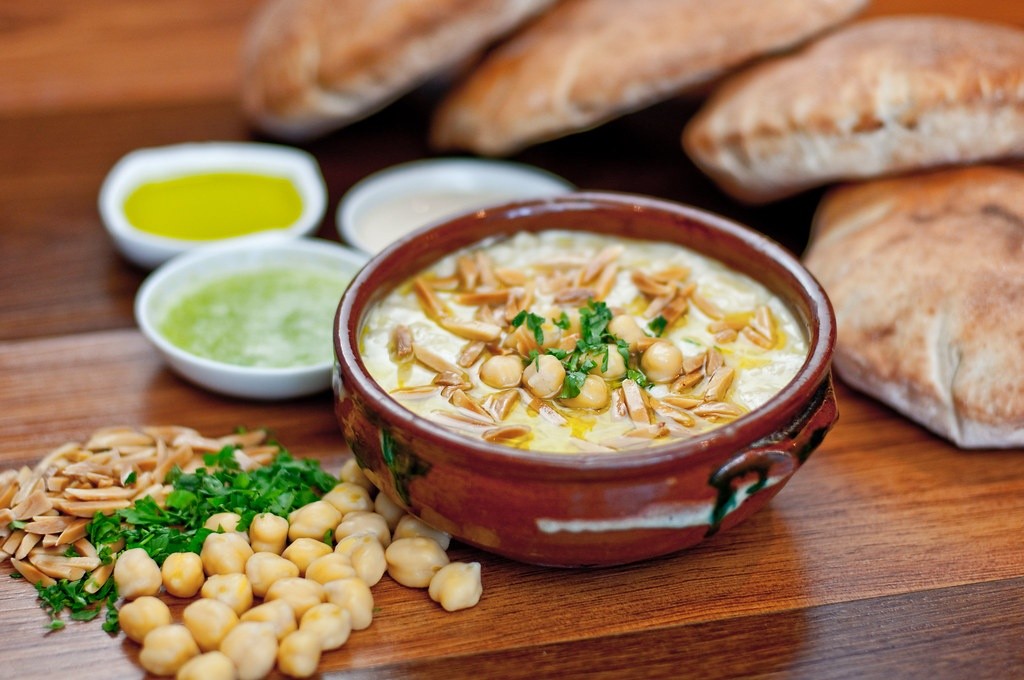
top-left (226, 0), bottom-right (1024, 201)
top-left (799, 156), bottom-right (1024, 450)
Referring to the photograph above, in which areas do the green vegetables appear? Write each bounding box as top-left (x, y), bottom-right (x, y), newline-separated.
top-left (6, 421), bottom-right (342, 636)
top-left (508, 297), bottom-right (667, 402)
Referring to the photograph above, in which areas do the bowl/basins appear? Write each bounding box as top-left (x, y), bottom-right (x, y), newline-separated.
top-left (337, 157), bottom-right (577, 260)
top-left (134, 236), bottom-right (372, 397)
top-left (332, 191), bottom-right (840, 567)
top-left (96, 144), bottom-right (328, 268)
